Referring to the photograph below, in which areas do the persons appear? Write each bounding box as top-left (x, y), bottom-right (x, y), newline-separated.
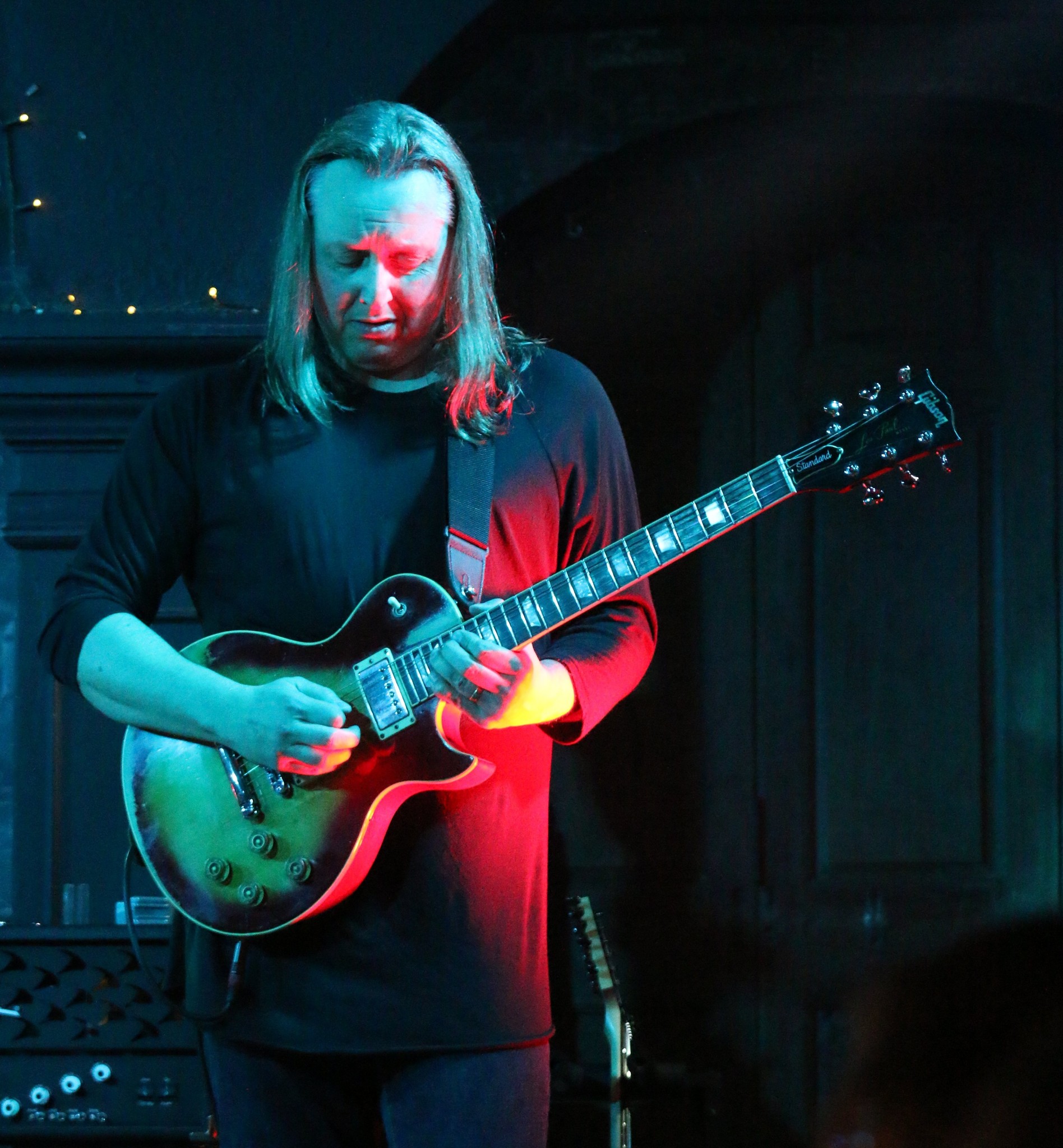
top-left (816, 911), bottom-right (1063, 1148)
top-left (41, 99), bottom-right (662, 1148)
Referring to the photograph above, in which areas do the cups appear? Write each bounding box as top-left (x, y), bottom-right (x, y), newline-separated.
top-left (61, 882), bottom-right (91, 925)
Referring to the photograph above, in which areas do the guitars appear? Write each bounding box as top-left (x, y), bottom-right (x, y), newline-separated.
top-left (117, 360), bottom-right (973, 942)
top-left (563, 894), bottom-right (640, 1147)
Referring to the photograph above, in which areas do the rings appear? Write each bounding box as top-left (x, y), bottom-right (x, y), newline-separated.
top-left (468, 687), bottom-right (485, 703)
